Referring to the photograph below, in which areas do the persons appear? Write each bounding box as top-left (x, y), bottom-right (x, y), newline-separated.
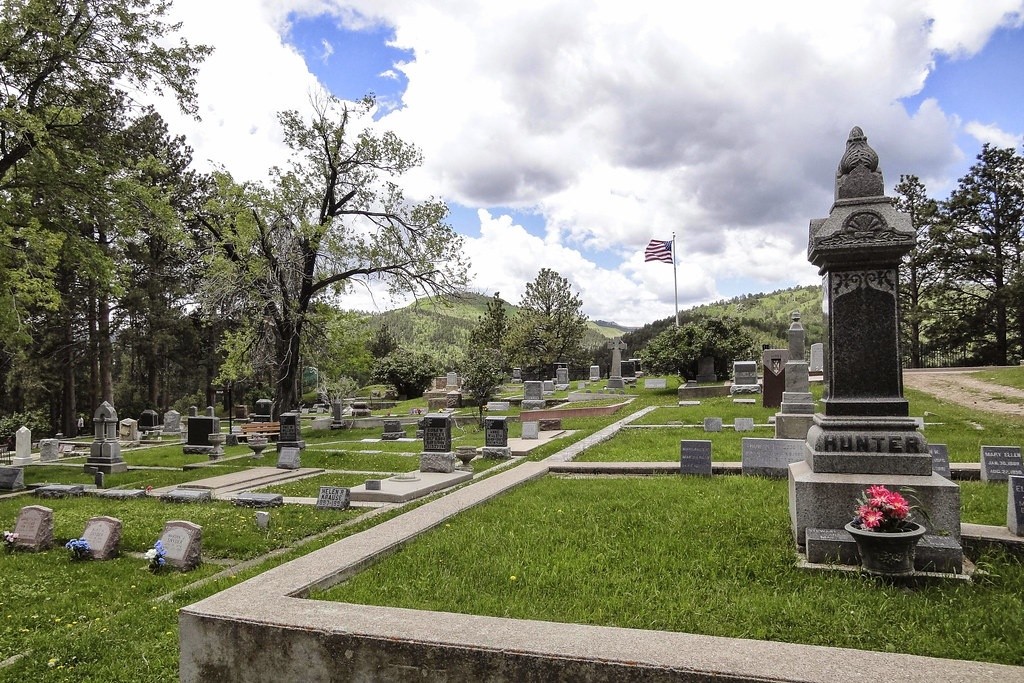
top-left (645, 240), bottom-right (673, 263)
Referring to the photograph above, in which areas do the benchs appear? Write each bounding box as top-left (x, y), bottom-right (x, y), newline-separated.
top-left (235, 422), bottom-right (280, 440)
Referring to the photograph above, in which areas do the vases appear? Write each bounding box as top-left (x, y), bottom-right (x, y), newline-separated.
top-left (845, 521), bottom-right (926, 576)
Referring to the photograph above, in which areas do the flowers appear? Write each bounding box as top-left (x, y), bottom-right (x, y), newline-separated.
top-left (854, 485), bottom-right (933, 529)
